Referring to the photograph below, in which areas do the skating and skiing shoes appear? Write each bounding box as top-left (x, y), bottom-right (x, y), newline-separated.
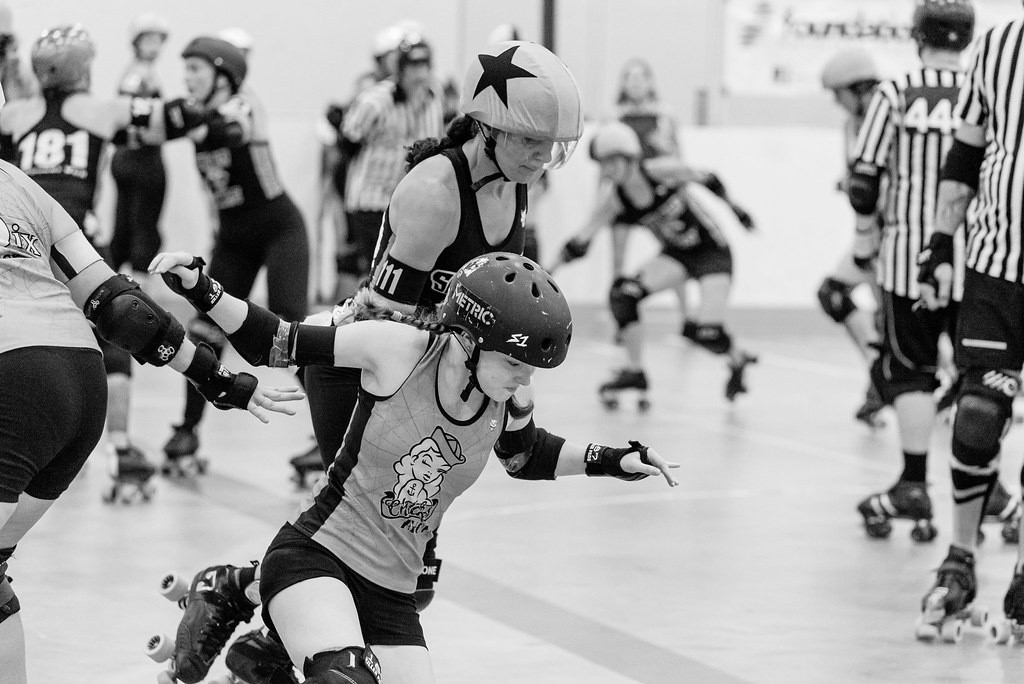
top-left (990, 566), bottom-right (1024, 645)
top-left (599, 366), bottom-right (650, 413)
top-left (913, 556), bottom-right (988, 644)
top-left (720, 349), bottom-right (761, 403)
top-left (99, 444), bottom-right (158, 506)
top-left (856, 383), bottom-right (892, 429)
top-left (287, 445), bottom-right (328, 490)
top-left (208, 624), bottom-right (300, 684)
top-left (160, 423), bottom-right (209, 480)
top-left (144, 564), bottom-right (257, 684)
top-left (975, 481), bottom-right (1021, 544)
top-left (858, 479), bottom-right (937, 542)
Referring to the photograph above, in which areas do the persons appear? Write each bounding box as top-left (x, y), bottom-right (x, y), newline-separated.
top-left (916, 19), bottom-right (1024, 653)
top-left (0, 155), bottom-right (305, 684)
top-left (146, 250), bottom-right (679, 684)
top-left (0, 1), bottom-right (1024, 543)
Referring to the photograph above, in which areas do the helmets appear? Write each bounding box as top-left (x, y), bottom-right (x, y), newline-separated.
top-left (588, 119), bottom-right (646, 162)
top-left (371, 24), bottom-right (428, 57)
top-left (218, 30), bottom-right (256, 57)
top-left (31, 26), bottom-right (96, 92)
top-left (458, 40), bottom-right (586, 170)
top-left (910, 0), bottom-right (974, 53)
top-left (823, 46), bottom-right (886, 87)
top-left (432, 250), bottom-right (573, 369)
top-left (128, 16), bottom-right (167, 46)
top-left (185, 36), bottom-right (245, 93)
